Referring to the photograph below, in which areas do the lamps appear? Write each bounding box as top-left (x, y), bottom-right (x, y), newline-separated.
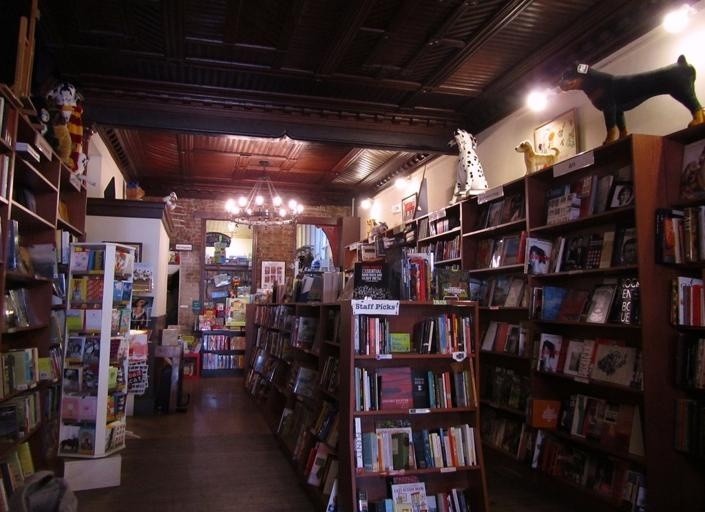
top-left (225, 161), bottom-right (303, 230)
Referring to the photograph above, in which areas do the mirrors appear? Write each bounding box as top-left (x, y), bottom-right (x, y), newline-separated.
top-left (201, 219), bottom-right (253, 267)
top-left (292, 217), bottom-right (345, 271)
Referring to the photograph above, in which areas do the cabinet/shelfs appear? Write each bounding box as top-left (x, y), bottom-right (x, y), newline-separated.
top-left (417, 203), bottom-right (462, 299)
top-left (0, 83), bottom-right (86, 510)
top-left (526, 133), bottom-right (681, 512)
top-left (353, 299), bottom-right (491, 512)
top-left (663, 122), bottom-right (705, 512)
top-left (58, 241), bottom-right (136, 459)
top-left (200, 267), bottom-right (257, 316)
top-left (356, 220), bottom-right (417, 262)
top-left (193, 318), bottom-right (246, 377)
top-left (460, 176), bottom-right (531, 497)
top-left (242, 300), bottom-right (344, 512)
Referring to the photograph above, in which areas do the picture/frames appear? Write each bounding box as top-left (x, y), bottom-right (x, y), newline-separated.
top-left (401, 192), bottom-right (418, 222)
top-left (532, 107), bottom-right (578, 171)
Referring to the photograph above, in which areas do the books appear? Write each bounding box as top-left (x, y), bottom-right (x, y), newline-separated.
top-left (466, 173), bottom-right (645, 511)
top-left (382, 209), bottom-right (472, 301)
top-left (201, 295), bottom-right (248, 369)
top-left (245, 273), bottom-right (477, 511)
top-left (654, 209), bottom-right (705, 455)
top-left (2, 105), bottom-right (64, 509)
top-left (55, 227), bottom-right (148, 453)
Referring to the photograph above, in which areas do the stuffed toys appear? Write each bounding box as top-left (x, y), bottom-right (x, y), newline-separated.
top-left (36, 80), bottom-right (97, 188)
